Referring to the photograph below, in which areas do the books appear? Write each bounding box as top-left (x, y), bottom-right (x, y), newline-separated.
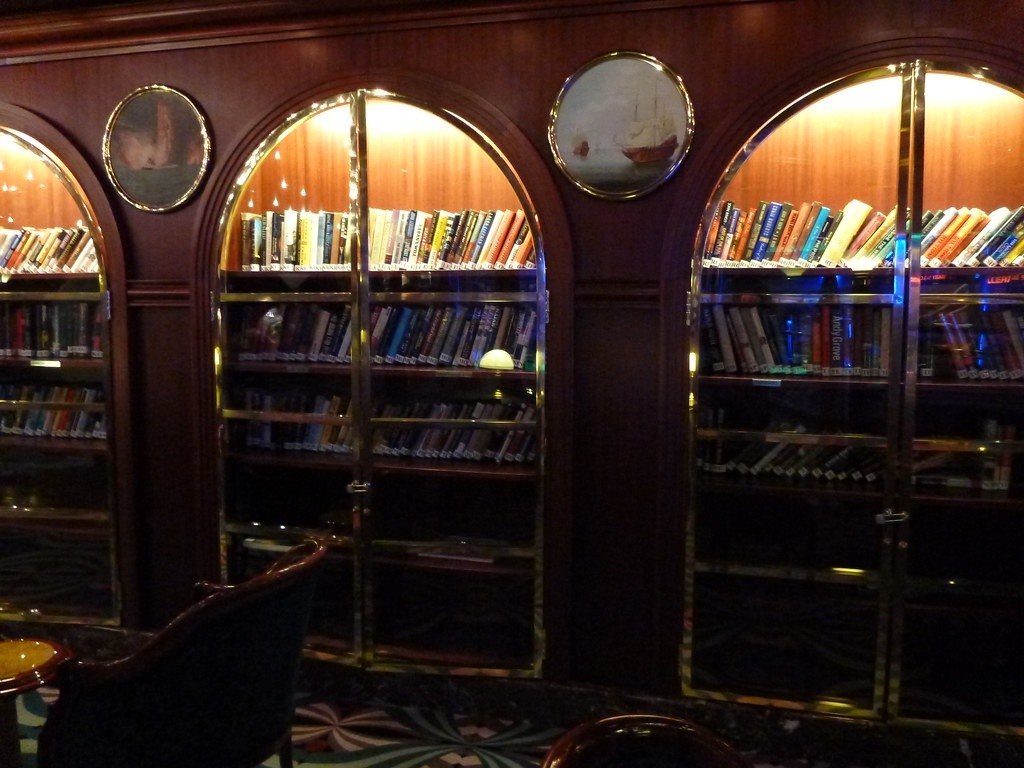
top-left (368, 392), bottom-right (540, 464)
top-left (238, 207), bottom-right (354, 270)
top-left (227, 297), bottom-right (355, 365)
top-left (699, 299), bottom-right (893, 381)
top-left (227, 374), bottom-right (357, 456)
top-left (0, 374), bottom-right (110, 439)
top-left (704, 194), bottom-right (911, 272)
top-left (920, 207), bottom-right (1024, 268)
top-left (0, 282), bottom-right (104, 360)
top-left (0, 222), bottom-right (103, 275)
top-left (919, 303), bottom-right (1024, 383)
top-left (370, 301), bottom-right (538, 372)
top-left (367, 207), bottom-right (544, 270)
top-left (693, 396), bottom-right (1023, 504)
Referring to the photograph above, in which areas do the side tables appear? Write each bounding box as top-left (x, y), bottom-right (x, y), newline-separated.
top-left (0, 638), bottom-right (75, 768)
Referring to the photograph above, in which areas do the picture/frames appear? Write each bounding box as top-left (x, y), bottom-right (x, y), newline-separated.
top-left (547, 49), bottom-right (697, 202)
top-left (101, 84), bottom-right (213, 213)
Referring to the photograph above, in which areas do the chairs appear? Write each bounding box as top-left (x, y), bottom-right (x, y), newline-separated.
top-left (540, 714), bottom-right (756, 768)
top-left (36, 534), bottom-right (328, 768)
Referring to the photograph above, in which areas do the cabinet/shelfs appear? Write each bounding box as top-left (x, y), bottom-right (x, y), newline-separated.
top-left (0, 223), bottom-right (119, 626)
top-left (201, 206), bottom-right (546, 681)
top-left (680, 201), bottom-right (1024, 713)
